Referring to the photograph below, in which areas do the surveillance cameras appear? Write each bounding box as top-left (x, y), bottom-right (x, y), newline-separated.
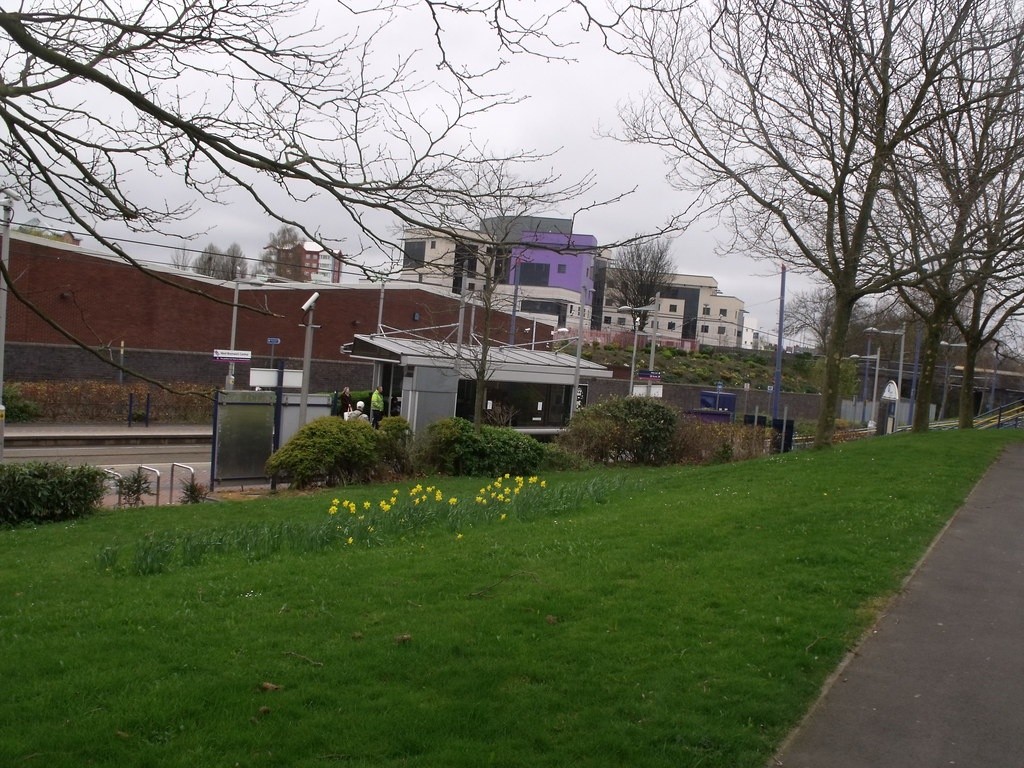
top-left (635, 318), bottom-right (640, 323)
top-left (301, 292), bottom-right (319, 311)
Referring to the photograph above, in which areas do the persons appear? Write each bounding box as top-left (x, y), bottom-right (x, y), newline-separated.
top-left (391, 397), bottom-right (399, 417)
top-left (341, 387), bottom-right (352, 413)
top-left (347, 401), bottom-right (368, 420)
top-left (370, 386), bottom-right (384, 430)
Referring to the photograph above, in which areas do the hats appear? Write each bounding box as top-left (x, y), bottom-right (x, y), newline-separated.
top-left (357, 401), bottom-right (364, 409)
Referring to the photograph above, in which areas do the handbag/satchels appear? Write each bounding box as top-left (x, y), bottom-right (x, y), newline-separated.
top-left (344, 406), bottom-right (353, 421)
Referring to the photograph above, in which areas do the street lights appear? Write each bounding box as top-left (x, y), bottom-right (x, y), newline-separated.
top-left (863, 328), bottom-right (906, 434)
top-left (226, 278), bottom-right (266, 389)
top-left (618, 305), bottom-right (638, 397)
top-left (849, 354), bottom-right (880, 433)
top-left (301, 292), bottom-right (320, 435)
top-left (569, 287), bottom-right (597, 422)
